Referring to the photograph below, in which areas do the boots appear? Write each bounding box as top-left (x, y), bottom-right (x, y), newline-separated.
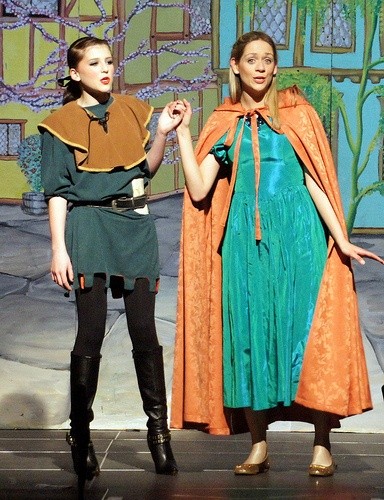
top-left (66, 352), bottom-right (102, 478)
top-left (131, 346), bottom-right (179, 475)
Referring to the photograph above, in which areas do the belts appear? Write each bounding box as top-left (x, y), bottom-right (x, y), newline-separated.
top-left (74, 197), bottom-right (147, 210)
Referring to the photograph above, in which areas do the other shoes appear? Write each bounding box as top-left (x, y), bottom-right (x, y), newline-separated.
top-left (234, 453), bottom-right (270, 475)
top-left (310, 454), bottom-right (336, 476)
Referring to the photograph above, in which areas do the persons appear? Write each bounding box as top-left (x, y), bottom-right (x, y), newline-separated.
top-left (37, 36), bottom-right (186, 479)
top-left (169, 32), bottom-right (384, 477)
